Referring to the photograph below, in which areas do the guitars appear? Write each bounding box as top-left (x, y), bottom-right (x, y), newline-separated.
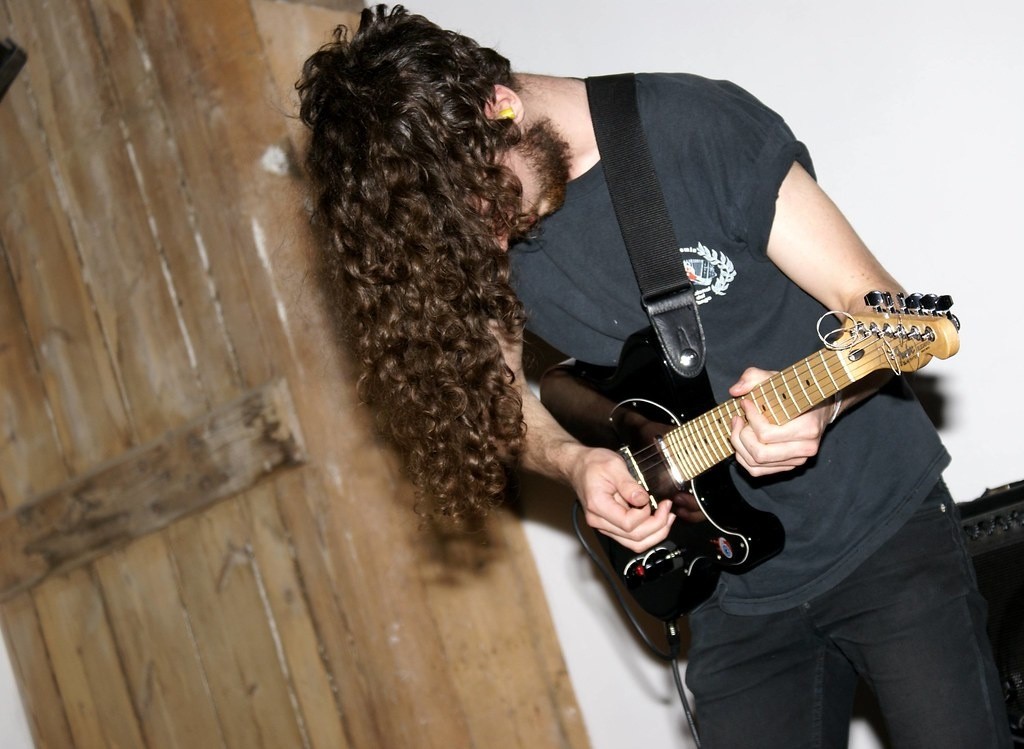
top-left (571, 289), bottom-right (964, 626)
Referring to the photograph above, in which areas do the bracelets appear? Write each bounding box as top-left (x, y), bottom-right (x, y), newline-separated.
top-left (829, 390), bottom-right (844, 423)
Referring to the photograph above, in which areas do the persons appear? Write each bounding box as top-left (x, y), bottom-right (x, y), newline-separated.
top-left (291, 3), bottom-right (1011, 749)
